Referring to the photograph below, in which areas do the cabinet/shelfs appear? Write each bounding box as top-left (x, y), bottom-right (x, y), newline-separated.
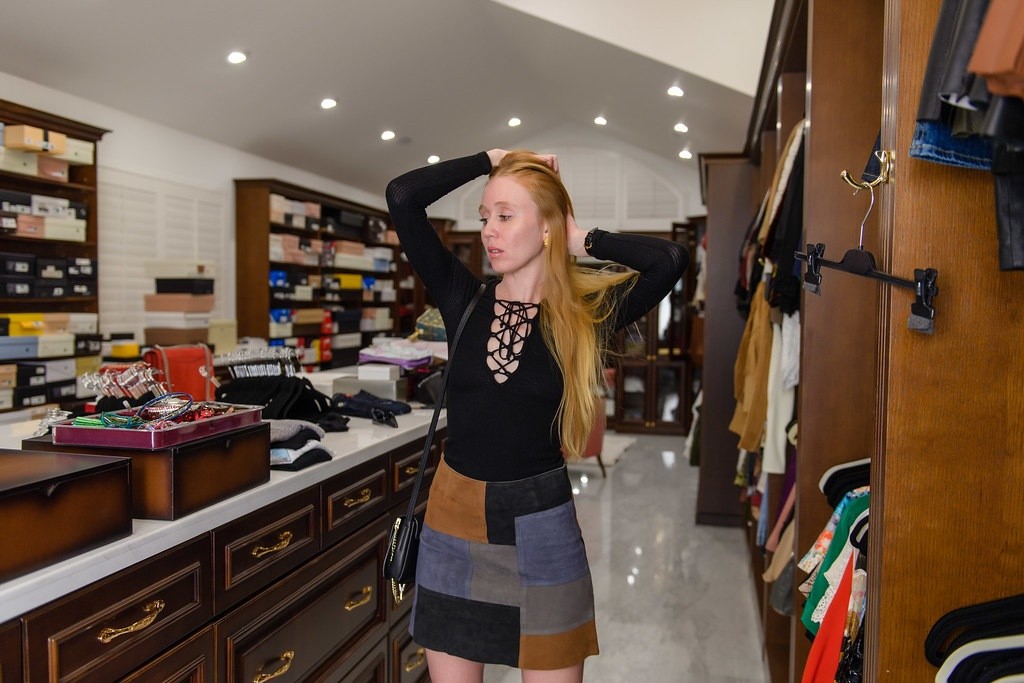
top-left (233, 178), bottom-right (425, 373)
top-left (695, 0), bottom-right (1024, 683)
top-left (0, 406), bottom-right (447, 683)
top-left (0, 99), bottom-right (113, 415)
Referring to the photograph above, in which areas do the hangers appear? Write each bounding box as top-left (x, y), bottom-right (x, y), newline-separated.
top-left (790, 183), bottom-right (937, 335)
top-left (922, 593), bottom-right (1024, 683)
top-left (818, 458), bottom-right (869, 554)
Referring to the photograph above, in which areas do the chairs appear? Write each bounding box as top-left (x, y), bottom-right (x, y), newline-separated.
top-left (140, 342), bottom-right (216, 403)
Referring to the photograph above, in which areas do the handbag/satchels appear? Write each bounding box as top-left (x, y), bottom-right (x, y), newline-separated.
top-left (382, 501), bottom-right (430, 583)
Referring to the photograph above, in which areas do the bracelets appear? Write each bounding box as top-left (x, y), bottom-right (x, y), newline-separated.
top-left (583, 227), bottom-right (598, 257)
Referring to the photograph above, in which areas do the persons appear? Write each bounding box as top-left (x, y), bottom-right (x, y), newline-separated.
top-left (385, 148), bottom-right (690, 683)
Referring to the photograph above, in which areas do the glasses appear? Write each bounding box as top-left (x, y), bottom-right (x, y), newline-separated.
top-left (370, 406), bottom-right (398, 429)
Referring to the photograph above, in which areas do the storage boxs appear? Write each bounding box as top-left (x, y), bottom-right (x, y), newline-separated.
top-left (0, 189), bottom-right (215, 411)
top-left (21, 423), bottom-right (272, 522)
top-left (0, 145), bottom-right (38, 175)
top-left (0, 448), bottom-right (133, 583)
top-left (3, 125), bottom-right (67, 155)
top-left (36, 151), bottom-right (68, 181)
top-left (49, 136), bottom-right (94, 165)
